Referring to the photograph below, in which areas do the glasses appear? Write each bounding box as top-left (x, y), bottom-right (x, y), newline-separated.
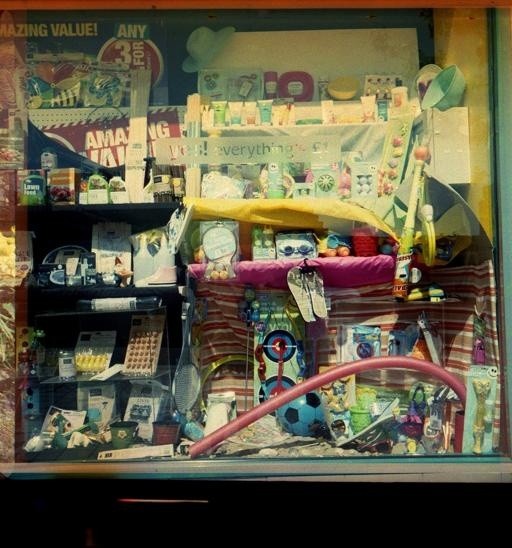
top-left (279, 245), bottom-right (314, 256)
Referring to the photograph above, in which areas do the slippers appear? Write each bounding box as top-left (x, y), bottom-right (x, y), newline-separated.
top-left (287, 266), bottom-right (318, 322)
top-left (302, 269), bottom-right (329, 318)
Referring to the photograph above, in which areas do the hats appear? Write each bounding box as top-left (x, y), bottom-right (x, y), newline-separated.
top-left (183, 26), bottom-right (234, 73)
